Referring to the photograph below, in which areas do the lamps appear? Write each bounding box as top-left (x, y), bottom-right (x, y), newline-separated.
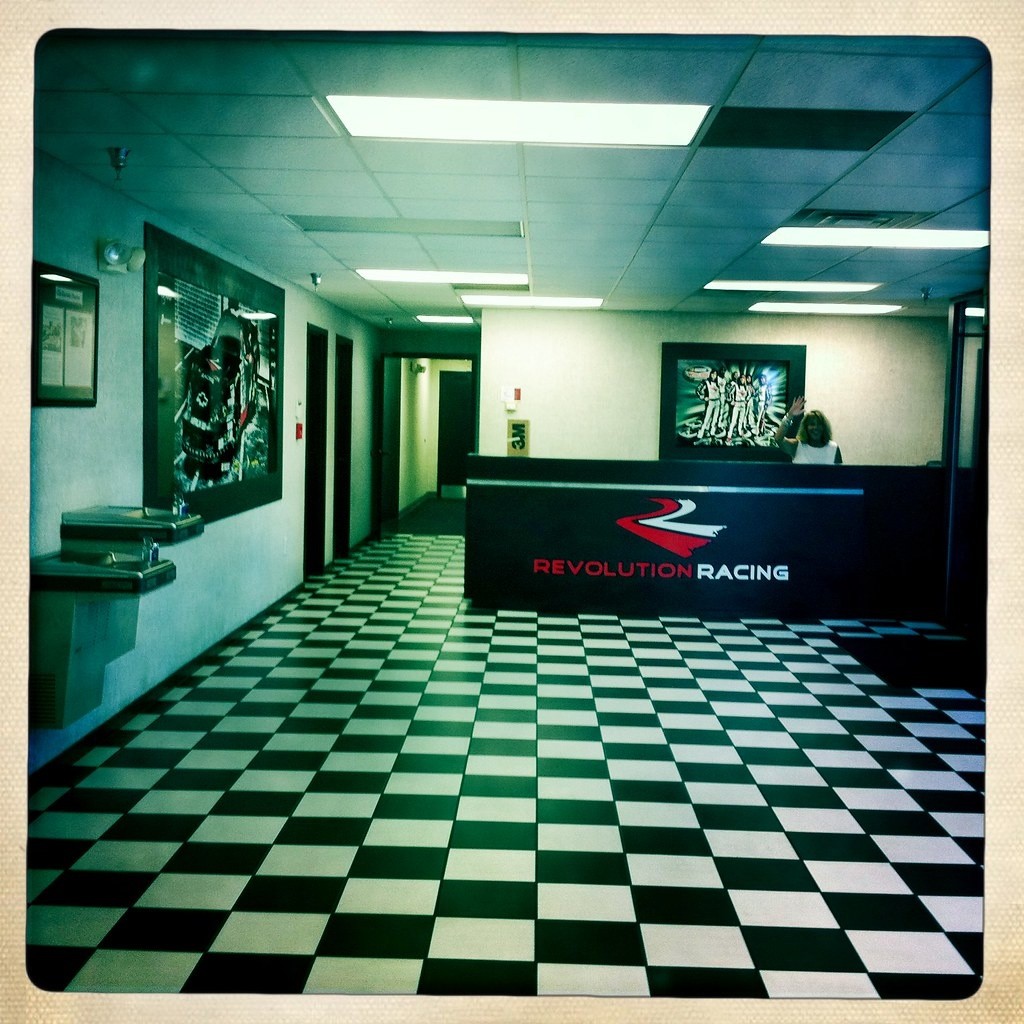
top-left (97, 236), bottom-right (147, 276)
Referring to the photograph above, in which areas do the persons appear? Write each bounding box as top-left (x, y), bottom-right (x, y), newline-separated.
top-left (775, 396), bottom-right (843, 463)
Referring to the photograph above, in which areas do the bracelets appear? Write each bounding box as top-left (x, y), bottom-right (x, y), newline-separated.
top-left (782, 416), bottom-right (794, 426)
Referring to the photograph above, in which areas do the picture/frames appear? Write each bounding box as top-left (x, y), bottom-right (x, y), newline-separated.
top-left (659, 341), bottom-right (806, 462)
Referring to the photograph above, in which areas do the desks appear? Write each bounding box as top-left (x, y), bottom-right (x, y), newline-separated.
top-left (63, 504), bottom-right (204, 552)
top-left (29, 552), bottom-right (176, 728)
